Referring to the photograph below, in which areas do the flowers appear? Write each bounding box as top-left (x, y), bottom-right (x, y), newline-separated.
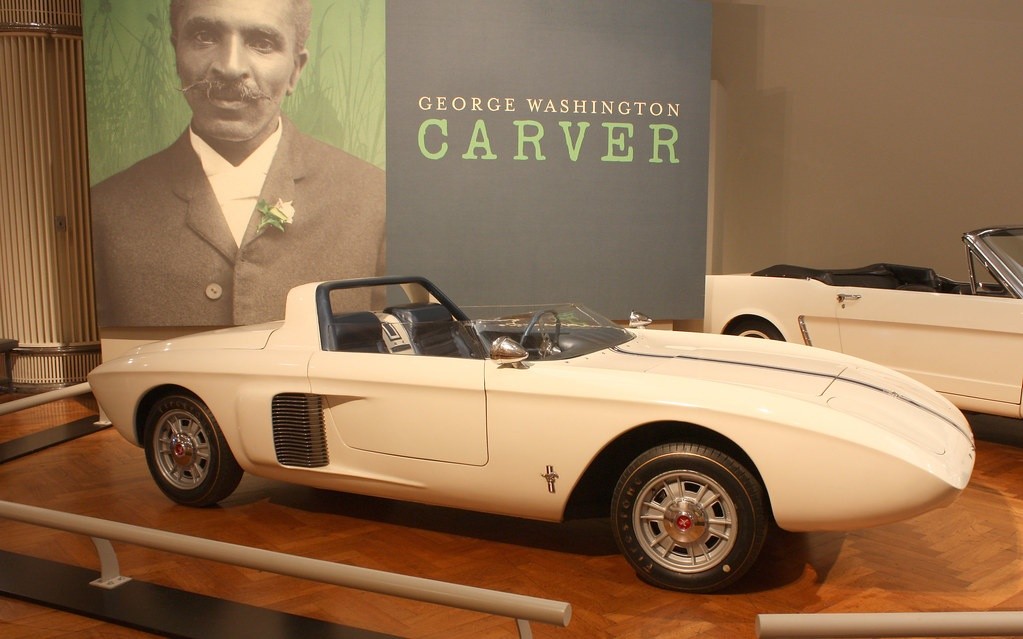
top-left (254, 198), bottom-right (295, 234)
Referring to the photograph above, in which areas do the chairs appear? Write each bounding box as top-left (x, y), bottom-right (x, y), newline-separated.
top-left (384, 301), bottom-right (484, 358)
top-left (333, 311), bottom-right (391, 354)
top-left (887, 263), bottom-right (943, 293)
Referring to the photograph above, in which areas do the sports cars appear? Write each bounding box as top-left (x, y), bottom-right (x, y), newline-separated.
top-left (89, 273), bottom-right (976, 596)
top-left (702, 224), bottom-right (1023, 450)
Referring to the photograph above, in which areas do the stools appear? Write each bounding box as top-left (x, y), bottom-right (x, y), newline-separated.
top-left (0, 338), bottom-right (19, 393)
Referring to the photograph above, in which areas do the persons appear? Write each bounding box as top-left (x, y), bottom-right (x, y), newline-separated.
top-left (89, 2), bottom-right (384, 328)
